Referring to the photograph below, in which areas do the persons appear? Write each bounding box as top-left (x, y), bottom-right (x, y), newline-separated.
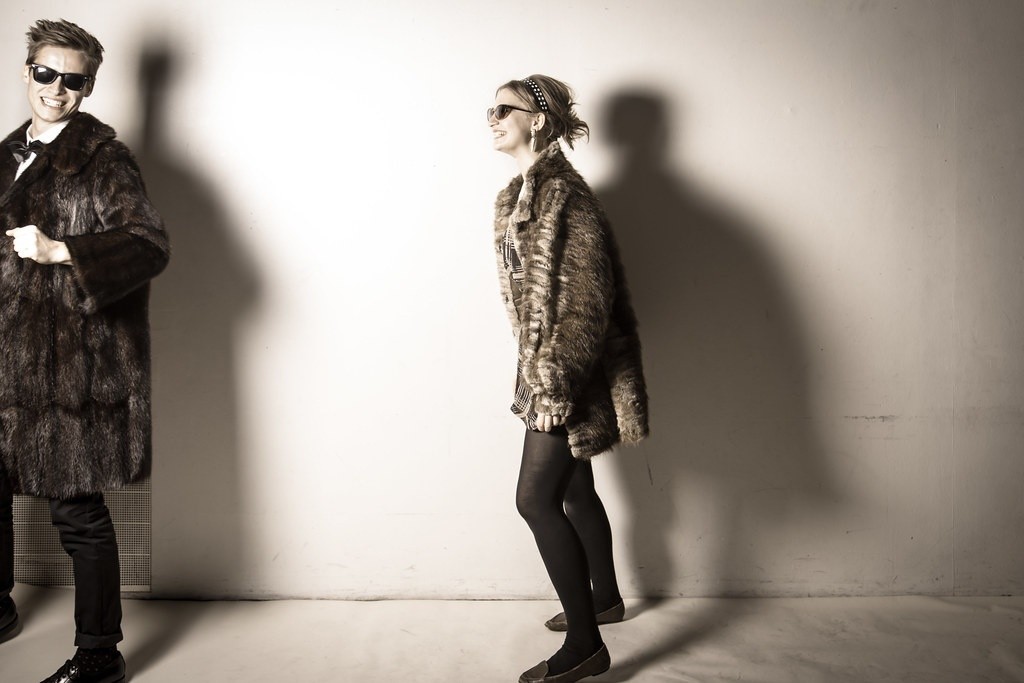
top-left (0, 19), bottom-right (171, 683)
top-left (486, 75), bottom-right (650, 683)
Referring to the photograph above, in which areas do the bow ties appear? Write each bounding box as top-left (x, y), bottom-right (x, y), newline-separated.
top-left (7, 140), bottom-right (45, 162)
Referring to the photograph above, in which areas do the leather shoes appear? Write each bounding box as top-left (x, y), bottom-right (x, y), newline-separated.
top-left (40, 651), bottom-right (127, 683)
top-left (0, 597), bottom-right (19, 637)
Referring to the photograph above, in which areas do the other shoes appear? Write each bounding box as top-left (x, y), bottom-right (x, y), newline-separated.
top-left (545, 598), bottom-right (625, 631)
top-left (518, 643), bottom-right (611, 683)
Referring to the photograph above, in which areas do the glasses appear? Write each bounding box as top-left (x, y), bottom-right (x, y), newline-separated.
top-left (31, 63), bottom-right (93, 91)
top-left (487, 104), bottom-right (539, 122)
